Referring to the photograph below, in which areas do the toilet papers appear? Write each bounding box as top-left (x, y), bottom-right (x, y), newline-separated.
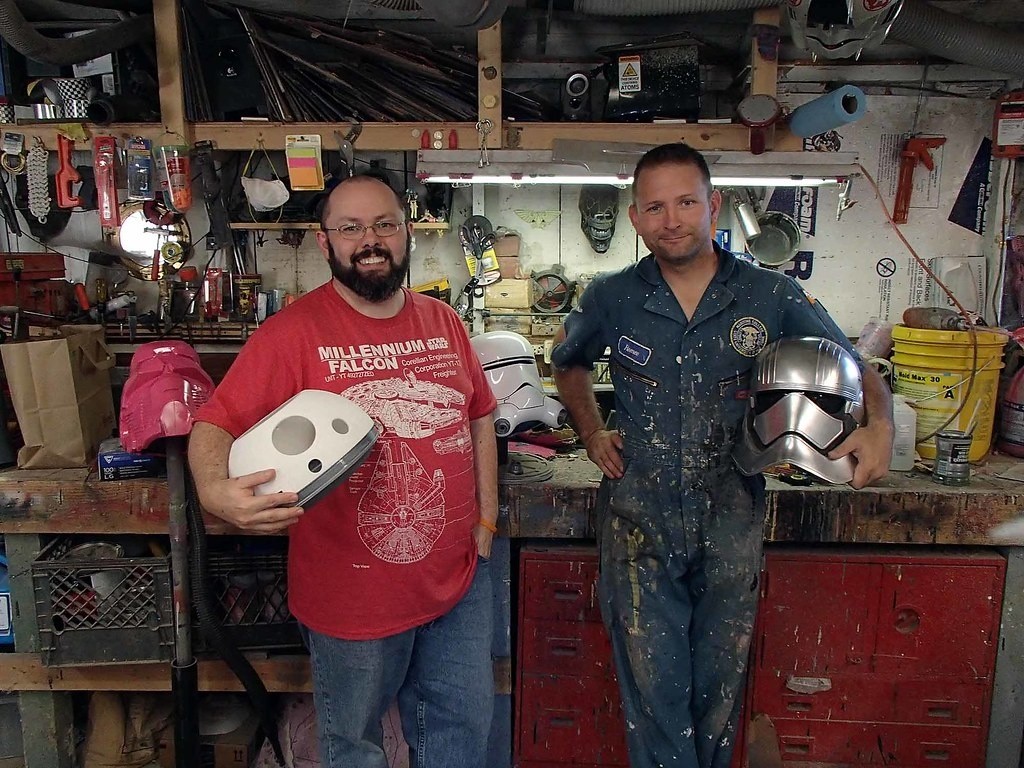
top-left (789, 84), bottom-right (867, 138)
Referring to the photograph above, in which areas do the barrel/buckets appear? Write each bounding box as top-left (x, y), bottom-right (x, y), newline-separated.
top-left (891, 323), bottom-right (1009, 461)
top-left (152, 132), bottom-right (193, 213)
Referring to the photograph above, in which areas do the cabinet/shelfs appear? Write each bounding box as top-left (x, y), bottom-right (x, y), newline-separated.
top-left (0, 467), bottom-right (319, 768)
top-left (1, 1), bottom-right (805, 156)
top-left (511, 547), bottom-right (1006, 768)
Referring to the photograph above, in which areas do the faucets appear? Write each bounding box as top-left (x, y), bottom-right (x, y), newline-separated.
top-left (835, 193), bottom-right (859, 221)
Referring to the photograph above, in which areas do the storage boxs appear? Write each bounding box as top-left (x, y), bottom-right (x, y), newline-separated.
top-left (194, 544), bottom-right (303, 653)
top-left (158, 708), bottom-right (265, 767)
top-left (98, 439), bottom-right (165, 479)
top-left (485, 236), bottom-right (538, 334)
top-left (30, 536), bottom-right (176, 667)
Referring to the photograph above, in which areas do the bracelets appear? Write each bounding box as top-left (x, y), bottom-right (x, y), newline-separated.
top-left (479, 517), bottom-right (497, 531)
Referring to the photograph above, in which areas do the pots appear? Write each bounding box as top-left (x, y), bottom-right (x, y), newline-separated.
top-left (743, 186), bottom-right (801, 265)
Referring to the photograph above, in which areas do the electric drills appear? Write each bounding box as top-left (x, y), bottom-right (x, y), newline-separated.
top-left (903, 307), bottom-right (1014, 339)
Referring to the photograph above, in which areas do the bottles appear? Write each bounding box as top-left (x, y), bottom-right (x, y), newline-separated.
top-left (448, 129), bottom-right (457, 149)
top-left (889, 394), bottom-right (916, 470)
top-left (421, 129), bottom-right (430, 150)
top-left (1000, 365), bottom-right (1024, 457)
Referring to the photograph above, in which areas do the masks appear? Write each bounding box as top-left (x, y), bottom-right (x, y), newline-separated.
top-left (241, 145), bottom-right (290, 224)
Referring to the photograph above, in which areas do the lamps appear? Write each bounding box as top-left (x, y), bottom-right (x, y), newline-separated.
top-left (416, 149), bottom-right (864, 188)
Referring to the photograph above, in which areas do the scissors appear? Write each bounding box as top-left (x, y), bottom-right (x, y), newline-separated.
top-left (457, 224), bottom-right (497, 283)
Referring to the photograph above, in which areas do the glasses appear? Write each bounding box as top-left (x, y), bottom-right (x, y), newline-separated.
top-left (320, 221), bottom-right (406, 240)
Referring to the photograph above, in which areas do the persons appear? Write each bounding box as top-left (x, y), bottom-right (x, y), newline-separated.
top-left (553, 141), bottom-right (896, 768)
top-left (189, 170), bottom-right (501, 768)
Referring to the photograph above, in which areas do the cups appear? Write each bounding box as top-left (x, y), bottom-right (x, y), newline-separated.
top-left (932, 430), bottom-right (973, 486)
top-left (853, 316), bottom-right (893, 358)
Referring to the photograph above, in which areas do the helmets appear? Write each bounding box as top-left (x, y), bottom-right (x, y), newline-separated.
top-left (728, 334), bottom-right (864, 484)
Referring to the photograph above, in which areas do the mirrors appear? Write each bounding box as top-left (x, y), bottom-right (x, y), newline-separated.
top-left (112, 199), bottom-right (191, 280)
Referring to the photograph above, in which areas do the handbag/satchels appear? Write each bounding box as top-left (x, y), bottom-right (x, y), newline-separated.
top-left (0, 325), bottom-right (120, 470)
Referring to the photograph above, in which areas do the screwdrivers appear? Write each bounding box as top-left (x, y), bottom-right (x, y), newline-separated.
top-left (96, 279), bottom-right (109, 327)
top-left (74, 283), bottom-right (94, 324)
top-left (760, 472), bottom-right (813, 487)
